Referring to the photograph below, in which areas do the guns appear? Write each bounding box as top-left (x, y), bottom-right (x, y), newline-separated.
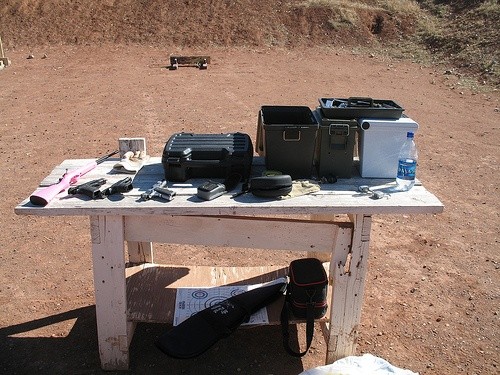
top-left (30, 151), bottom-right (119, 206)
top-left (141, 178), bottom-right (176, 201)
top-left (68, 177), bottom-right (106, 200)
top-left (111, 176), bottom-right (133, 192)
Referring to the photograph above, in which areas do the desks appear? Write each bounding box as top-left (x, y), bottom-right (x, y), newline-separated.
top-left (15, 159), bottom-right (446, 368)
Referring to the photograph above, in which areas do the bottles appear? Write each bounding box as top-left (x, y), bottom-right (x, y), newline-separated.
top-left (396, 131), bottom-right (418, 191)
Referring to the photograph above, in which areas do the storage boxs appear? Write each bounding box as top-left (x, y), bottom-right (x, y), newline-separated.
top-left (256, 105), bottom-right (318, 179)
top-left (318, 96), bottom-right (405, 120)
top-left (312, 109), bottom-right (358, 178)
top-left (162, 132), bottom-right (253, 182)
top-left (357, 113), bottom-right (418, 179)
